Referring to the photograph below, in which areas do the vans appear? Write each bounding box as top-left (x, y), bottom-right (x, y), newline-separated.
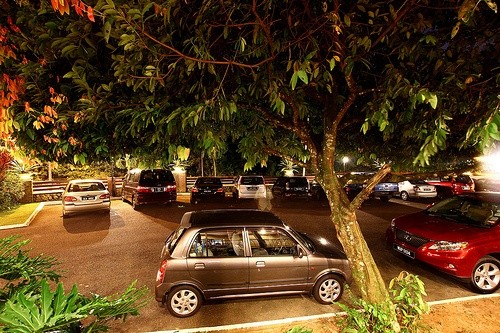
top-left (117, 165), bottom-right (178, 208)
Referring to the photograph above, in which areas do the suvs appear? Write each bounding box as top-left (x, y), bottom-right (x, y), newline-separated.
top-left (421, 172), bottom-right (476, 199)
top-left (385, 190), bottom-right (500, 295)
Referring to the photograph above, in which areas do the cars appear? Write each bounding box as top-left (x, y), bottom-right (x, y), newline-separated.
top-left (344, 176), bottom-right (397, 204)
top-left (231, 173), bottom-right (270, 203)
top-left (60, 176), bottom-right (111, 218)
top-left (188, 174), bottom-right (225, 207)
top-left (154, 208), bottom-right (353, 319)
top-left (397, 176), bottom-right (437, 200)
top-left (270, 175), bottom-right (314, 201)
